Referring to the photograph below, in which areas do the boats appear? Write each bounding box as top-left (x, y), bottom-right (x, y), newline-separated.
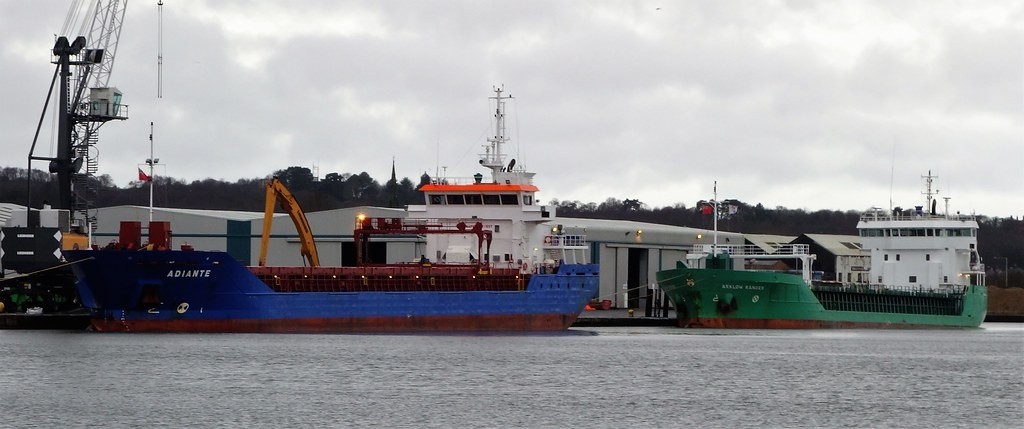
top-left (62, 218), bottom-right (599, 333)
top-left (656, 252), bottom-right (989, 329)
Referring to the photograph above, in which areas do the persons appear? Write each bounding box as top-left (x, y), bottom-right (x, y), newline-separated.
top-left (419, 254), bottom-right (427, 265)
top-left (507, 159), bottom-right (516, 172)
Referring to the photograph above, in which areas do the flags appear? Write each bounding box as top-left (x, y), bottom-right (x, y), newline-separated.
top-left (138, 168), bottom-right (152, 182)
top-left (728, 204), bottom-right (738, 215)
top-left (702, 204), bottom-right (714, 215)
